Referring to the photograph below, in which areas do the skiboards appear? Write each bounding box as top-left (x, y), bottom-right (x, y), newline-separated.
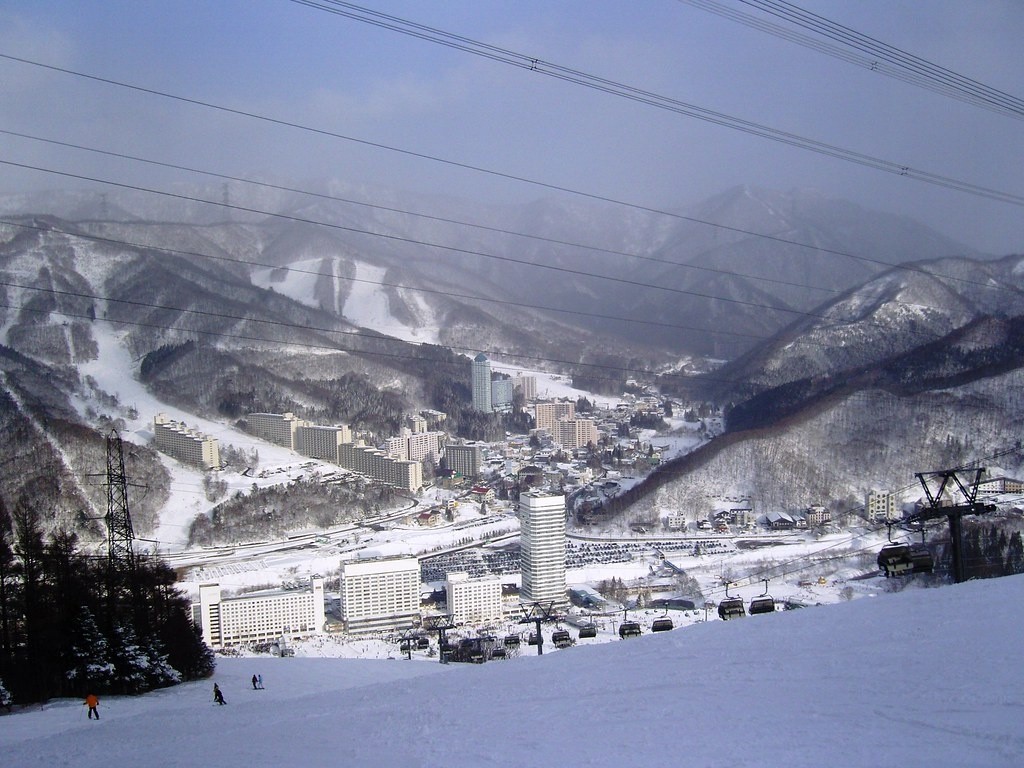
top-left (88, 716), bottom-right (99, 720)
top-left (252, 687), bottom-right (265, 690)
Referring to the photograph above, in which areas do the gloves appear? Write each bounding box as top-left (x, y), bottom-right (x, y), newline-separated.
top-left (97, 703), bottom-right (99, 705)
top-left (83, 703), bottom-right (86, 705)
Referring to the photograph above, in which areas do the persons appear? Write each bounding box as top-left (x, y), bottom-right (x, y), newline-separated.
top-left (213, 683), bottom-right (227, 705)
top-left (83, 692), bottom-right (99, 720)
top-left (252, 674), bottom-right (264, 690)
